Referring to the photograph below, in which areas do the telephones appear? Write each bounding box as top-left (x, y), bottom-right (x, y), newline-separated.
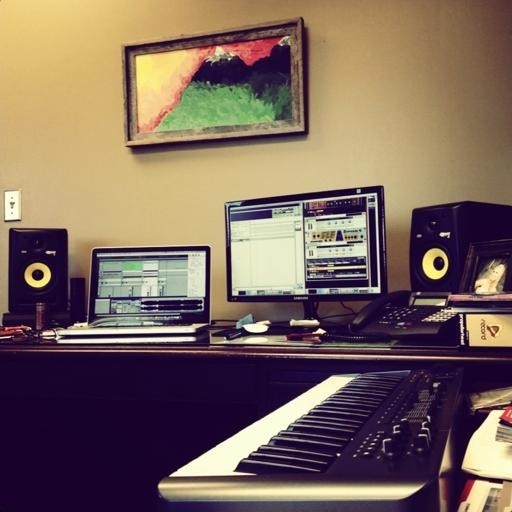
top-left (348, 290), bottom-right (460, 341)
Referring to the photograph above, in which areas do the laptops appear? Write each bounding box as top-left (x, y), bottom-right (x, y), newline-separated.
top-left (56, 245), bottom-right (212, 338)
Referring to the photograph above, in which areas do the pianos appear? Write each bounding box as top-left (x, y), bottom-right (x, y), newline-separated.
top-left (158, 366), bottom-right (485, 505)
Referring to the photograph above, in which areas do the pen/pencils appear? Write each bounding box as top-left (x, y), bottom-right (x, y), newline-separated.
top-left (475, 402), bottom-right (512, 415)
top-left (227, 330), bottom-right (245, 340)
top-left (286, 333), bottom-right (324, 341)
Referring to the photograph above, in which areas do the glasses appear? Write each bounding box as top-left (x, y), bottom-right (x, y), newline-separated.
top-left (12, 327), bottom-right (56, 343)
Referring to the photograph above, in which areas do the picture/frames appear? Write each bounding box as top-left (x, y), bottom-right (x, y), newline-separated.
top-left (458, 237), bottom-right (512, 295)
top-left (123, 18), bottom-right (308, 148)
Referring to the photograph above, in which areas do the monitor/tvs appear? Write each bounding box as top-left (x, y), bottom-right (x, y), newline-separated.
top-left (224, 185), bottom-right (387, 333)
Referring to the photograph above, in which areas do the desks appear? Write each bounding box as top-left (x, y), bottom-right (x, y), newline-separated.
top-left (0, 321), bottom-right (512, 401)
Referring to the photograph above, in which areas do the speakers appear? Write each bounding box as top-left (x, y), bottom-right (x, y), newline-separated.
top-left (410, 199), bottom-right (512, 296)
top-left (8, 226), bottom-right (70, 315)
